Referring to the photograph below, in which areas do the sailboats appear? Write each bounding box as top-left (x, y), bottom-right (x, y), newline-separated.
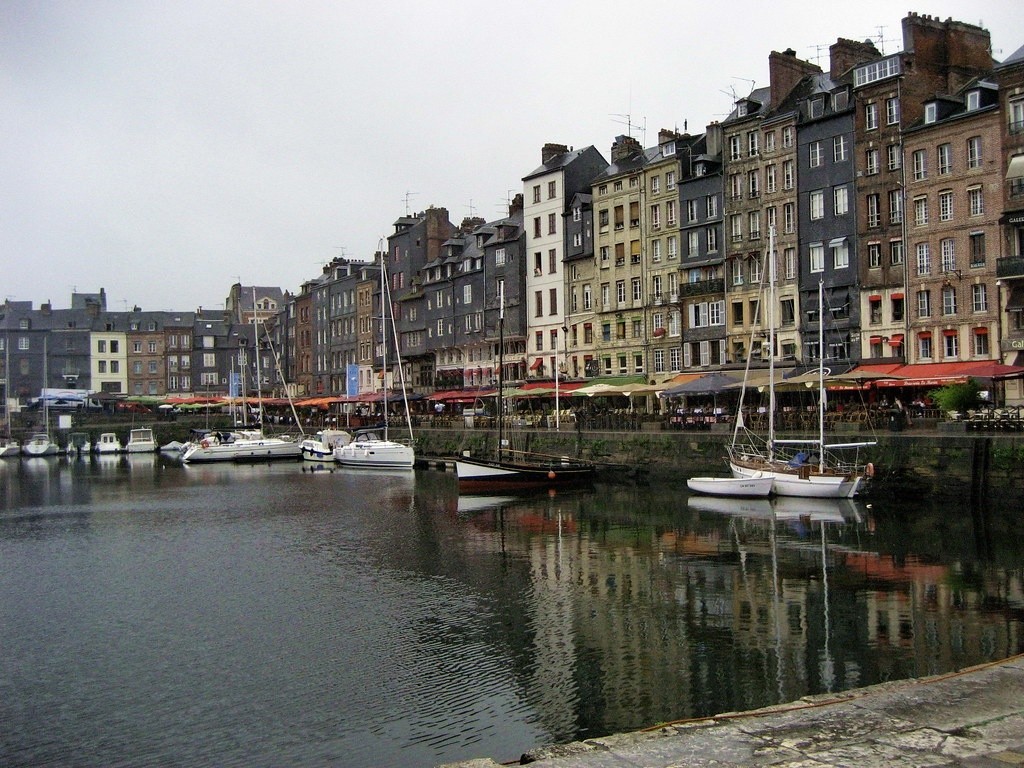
top-left (724, 224), bottom-right (878, 498)
top-left (0, 336), bottom-right (58, 458)
top-left (331, 238), bottom-right (416, 470)
top-left (180, 286), bottom-right (307, 459)
top-left (456, 281), bottom-right (596, 494)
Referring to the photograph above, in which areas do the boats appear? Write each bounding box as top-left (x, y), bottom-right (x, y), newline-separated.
top-left (687, 477), bottom-right (776, 496)
top-left (299, 430), bottom-right (351, 463)
top-left (66, 428), bottom-right (155, 456)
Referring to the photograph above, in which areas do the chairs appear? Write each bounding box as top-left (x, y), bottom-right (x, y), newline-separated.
top-left (384, 405), bottom-right (891, 430)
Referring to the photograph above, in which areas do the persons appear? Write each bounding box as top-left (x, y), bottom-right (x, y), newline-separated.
top-left (434, 402), bottom-right (445, 418)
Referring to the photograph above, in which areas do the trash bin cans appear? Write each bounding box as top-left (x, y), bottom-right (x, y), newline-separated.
top-left (889, 409), bottom-right (903, 432)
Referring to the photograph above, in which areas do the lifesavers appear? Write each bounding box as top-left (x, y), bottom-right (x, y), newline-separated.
top-left (866, 463), bottom-right (874, 477)
top-left (202, 441), bottom-right (208, 449)
